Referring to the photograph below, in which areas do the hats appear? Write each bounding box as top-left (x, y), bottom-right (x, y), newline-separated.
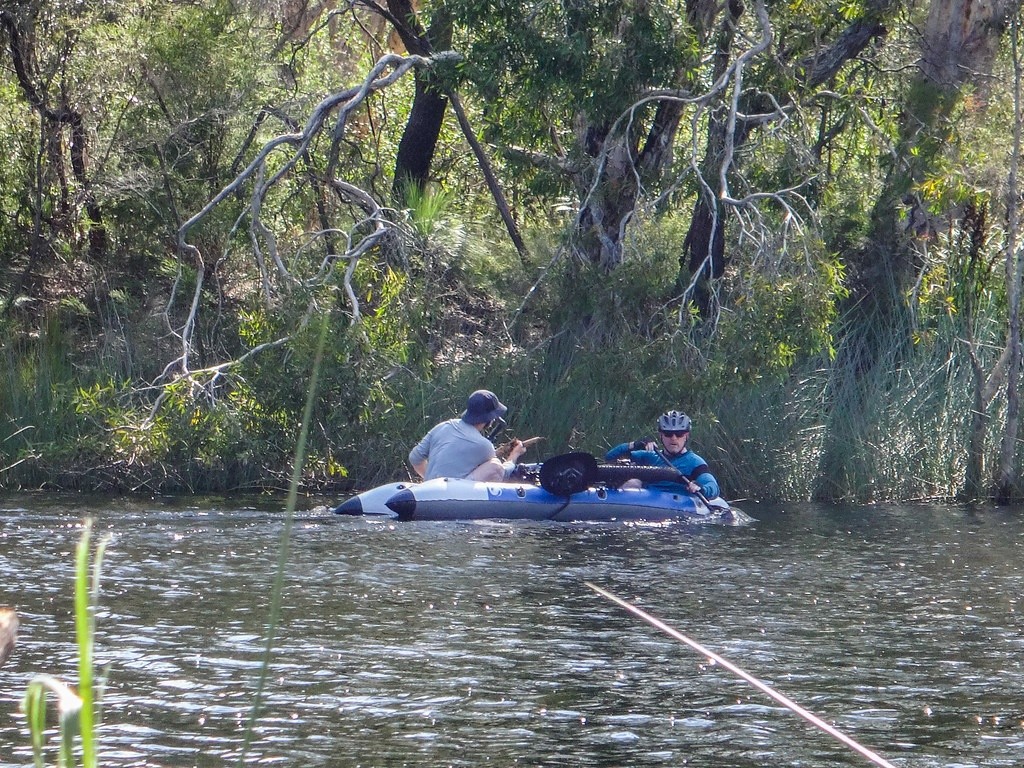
top-left (540, 452), bottom-right (598, 495)
top-left (460, 389), bottom-right (507, 426)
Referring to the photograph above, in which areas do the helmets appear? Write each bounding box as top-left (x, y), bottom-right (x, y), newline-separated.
top-left (656, 410), bottom-right (693, 432)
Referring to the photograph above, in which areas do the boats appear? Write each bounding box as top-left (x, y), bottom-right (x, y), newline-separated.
top-left (331, 450), bottom-right (736, 528)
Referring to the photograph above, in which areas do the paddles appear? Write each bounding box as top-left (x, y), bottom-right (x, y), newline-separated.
top-left (652, 443), bottom-right (732, 525)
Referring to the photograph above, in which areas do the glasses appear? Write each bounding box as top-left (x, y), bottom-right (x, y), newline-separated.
top-left (661, 431), bottom-right (687, 437)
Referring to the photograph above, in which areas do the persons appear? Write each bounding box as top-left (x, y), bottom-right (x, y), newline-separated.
top-left (409, 390), bottom-right (527, 483)
top-left (604, 410), bottom-right (720, 500)
